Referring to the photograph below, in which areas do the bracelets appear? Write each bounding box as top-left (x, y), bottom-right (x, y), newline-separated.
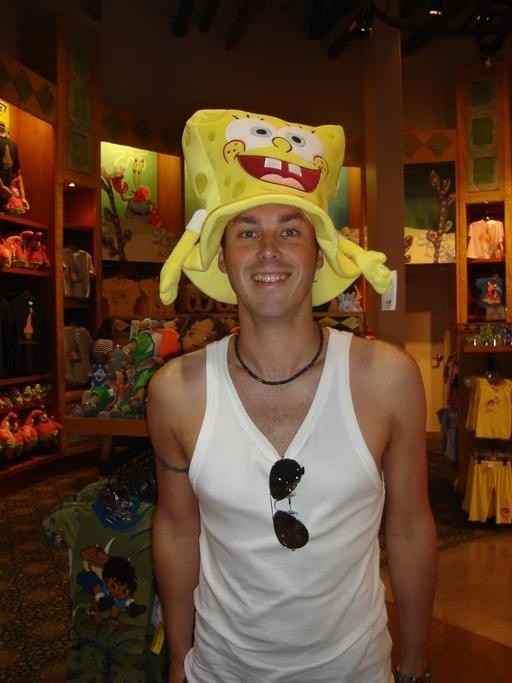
top-left (395, 663), bottom-right (433, 683)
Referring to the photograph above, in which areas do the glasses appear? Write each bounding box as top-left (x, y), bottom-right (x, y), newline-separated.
top-left (269, 459), bottom-right (309, 552)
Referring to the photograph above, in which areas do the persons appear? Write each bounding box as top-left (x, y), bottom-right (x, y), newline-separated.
top-left (145, 203), bottom-right (440, 683)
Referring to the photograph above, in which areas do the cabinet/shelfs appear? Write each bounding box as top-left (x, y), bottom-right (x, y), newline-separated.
top-left (458, 196), bottom-right (508, 343)
top-left (0, 99), bottom-right (60, 484)
top-left (59, 167), bottom-right (102, 401)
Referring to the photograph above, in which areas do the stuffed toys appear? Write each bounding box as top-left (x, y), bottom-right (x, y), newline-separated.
top-left (0, 380), bottom-right (64, 466)
top-left (76, 311), bottom-right (240, 419)
top-left (0, 121), bottom-right (29, 216)
top-left (0, 230), bottom-right (50, 268)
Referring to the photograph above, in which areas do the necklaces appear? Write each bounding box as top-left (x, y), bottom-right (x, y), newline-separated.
top-left (234, 320), bottom-right (323, 384)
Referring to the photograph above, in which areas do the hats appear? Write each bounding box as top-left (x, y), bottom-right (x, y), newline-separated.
top-left (159, 108), bottom-right (391, 308)
